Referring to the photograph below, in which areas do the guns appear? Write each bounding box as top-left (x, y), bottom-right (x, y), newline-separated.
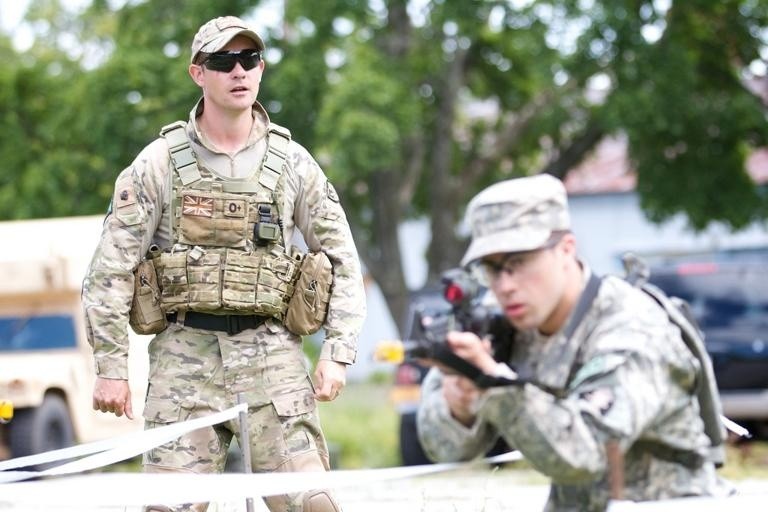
top-left (373, 269), bottom-right (516, 388)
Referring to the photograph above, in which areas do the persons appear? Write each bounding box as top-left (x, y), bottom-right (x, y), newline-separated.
top-left (82, 15), bottom-right (368, 512)
top-left (415, 173), bottom-right (735, 510)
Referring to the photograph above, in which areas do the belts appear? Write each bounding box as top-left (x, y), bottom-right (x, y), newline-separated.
top-left (166, 308), bottom-right (274, 335)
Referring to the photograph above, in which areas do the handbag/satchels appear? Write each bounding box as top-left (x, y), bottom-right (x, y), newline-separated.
top-left (284, 248), bottom-right (335, 336)
top-left (127, 261), bottom-right (165, 333)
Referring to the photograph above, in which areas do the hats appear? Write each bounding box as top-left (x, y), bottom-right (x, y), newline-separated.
top-left (191, 15), bottom-right (265, 64)
top-left (457, 173), bottom-right (571, 269)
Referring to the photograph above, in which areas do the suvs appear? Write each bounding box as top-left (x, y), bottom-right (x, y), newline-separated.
top-left (401, 182), bottom-right (768, 465)
top-left (1, 261), bottom-right (151, 471)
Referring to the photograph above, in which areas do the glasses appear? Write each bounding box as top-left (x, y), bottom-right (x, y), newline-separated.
top-left (468, 240), bottom-right (561, 289)
top-left (197, 47), bottom-right (262, 73)
